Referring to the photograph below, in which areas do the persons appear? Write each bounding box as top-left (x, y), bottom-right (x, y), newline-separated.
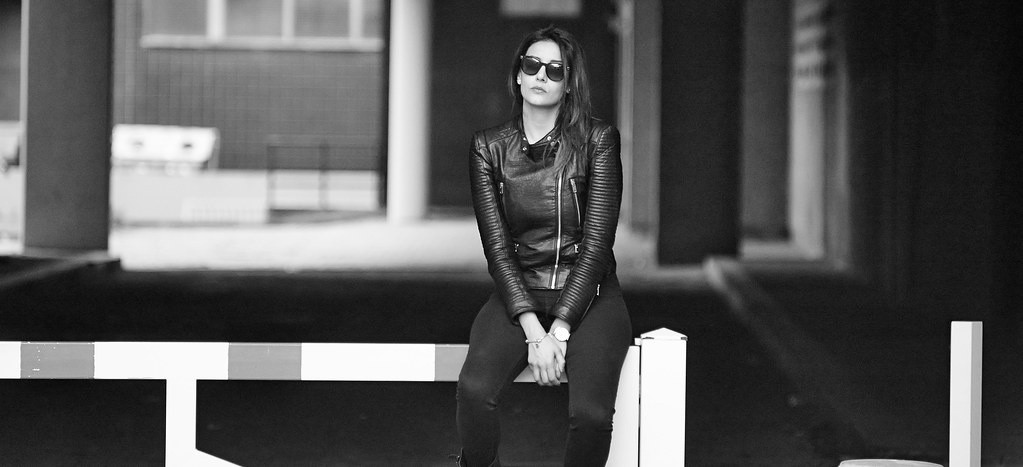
top-left (452, 26), bottom-right (633, 467)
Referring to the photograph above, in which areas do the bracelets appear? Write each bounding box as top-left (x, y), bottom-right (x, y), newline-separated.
top-left (524, 332), bottom-right (548, 348)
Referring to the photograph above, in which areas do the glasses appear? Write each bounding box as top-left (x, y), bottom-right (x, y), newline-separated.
top-left (520, 55), bottom-right (564, 81)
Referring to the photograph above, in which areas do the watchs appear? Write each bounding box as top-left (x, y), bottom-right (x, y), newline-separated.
top-left (548, 326), bottom-right (573, 343)
